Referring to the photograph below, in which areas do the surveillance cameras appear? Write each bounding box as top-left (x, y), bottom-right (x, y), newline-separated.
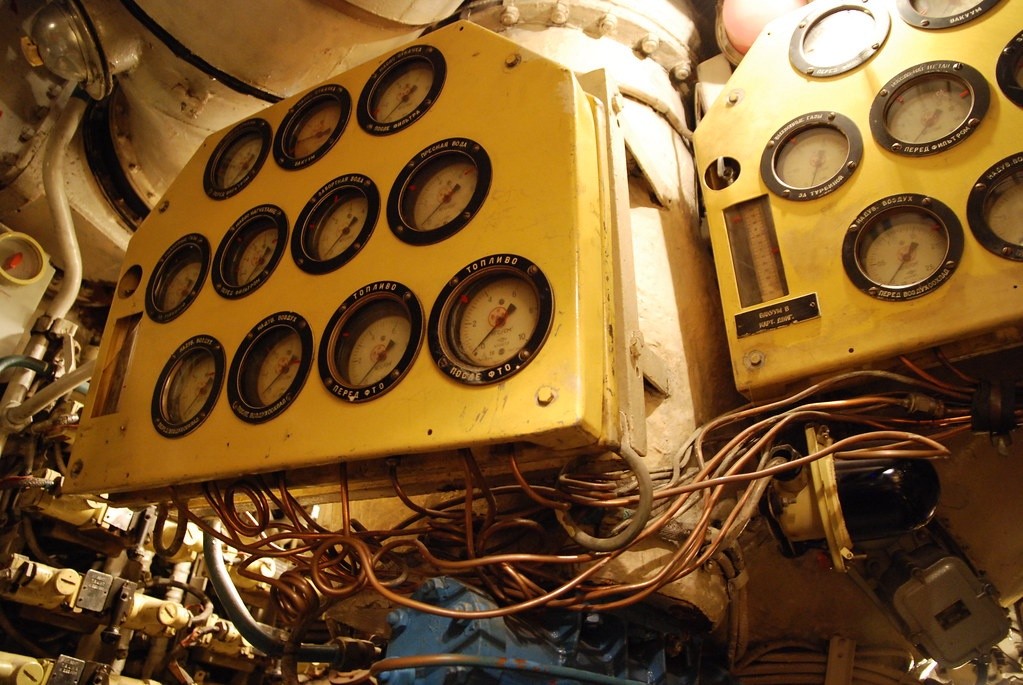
top-left (757, 455), bottom-right (941, 562)
